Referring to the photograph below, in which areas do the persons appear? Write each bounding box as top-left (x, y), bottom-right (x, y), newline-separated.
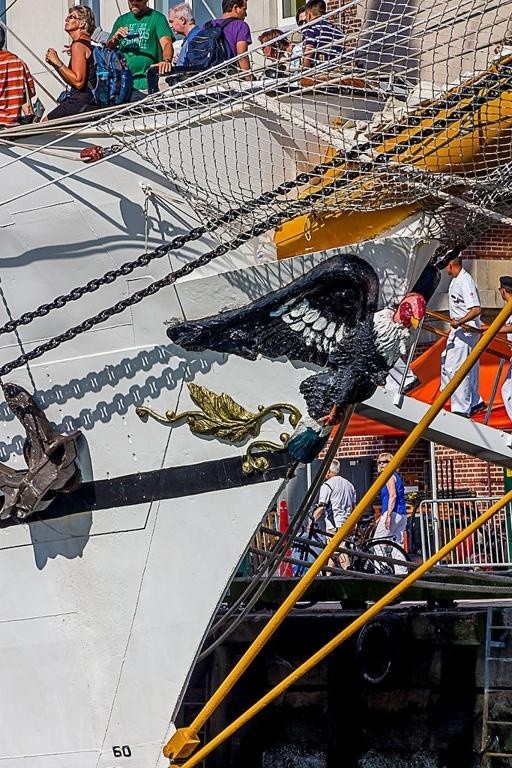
top-left (306, 459), bottom-right (357, 575)
top-left (445, 256), bottom-right (487, 419)
top-left (0, 27), bottom-right (35, 132)
top-left (384, 357), bottom-right (422, 396)
top-left (373, 453), bottom-right (408, 578)
top-left (483, 276), bottom-right (512, 423)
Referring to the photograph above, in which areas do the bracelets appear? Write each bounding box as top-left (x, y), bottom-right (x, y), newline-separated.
top-left (387, 512), bottom-right (391, 516)
top-left (457, 320), bottom-right (461, 326)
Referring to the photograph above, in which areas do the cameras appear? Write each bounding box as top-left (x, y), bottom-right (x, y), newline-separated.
top-left (124, 30), bottom-right (140, 39)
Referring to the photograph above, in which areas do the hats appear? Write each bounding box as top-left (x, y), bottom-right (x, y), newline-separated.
top-left (434, 247), bottom-right (462, 263)
top-left (499, 276), bottom-right (512, 287)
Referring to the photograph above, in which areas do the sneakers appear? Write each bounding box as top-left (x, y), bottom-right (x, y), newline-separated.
top-left (472, 402), bottom-right (487, 417)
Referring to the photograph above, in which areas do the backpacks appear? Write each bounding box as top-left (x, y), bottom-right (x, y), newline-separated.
top-left (70, 39), bottom-right (132, 107)
top-left (182, 17), bottom-right (238, 71)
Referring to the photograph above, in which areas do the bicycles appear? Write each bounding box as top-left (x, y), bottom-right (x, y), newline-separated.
top-left (269, 503), bottom-right (412, 608)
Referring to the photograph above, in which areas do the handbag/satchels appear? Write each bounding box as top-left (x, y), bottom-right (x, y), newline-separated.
top-left (18, 96), bottom-right (45, 126)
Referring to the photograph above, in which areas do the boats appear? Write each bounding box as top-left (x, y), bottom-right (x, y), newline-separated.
top-left (0, 0), bottom-right (511, 768)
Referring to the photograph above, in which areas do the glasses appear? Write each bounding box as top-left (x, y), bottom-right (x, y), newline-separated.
top-left (65, 15), bottom-right (81, 20)
top-left (378, 460), bottom-right (390, 465)
top-left (298, 18), bottom-right (306, 25)
